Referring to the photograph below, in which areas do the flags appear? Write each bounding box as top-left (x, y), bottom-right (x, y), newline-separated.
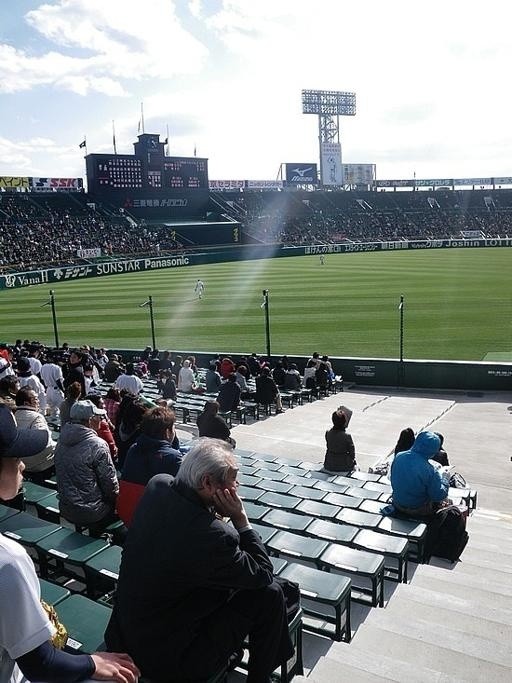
top-left (79, 135), bottom-right (87, 148)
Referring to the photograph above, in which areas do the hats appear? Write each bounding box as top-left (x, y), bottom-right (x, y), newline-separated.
top-left (1, 405), bottom-right (48, 457)
top-left (80, 343), bottom-right (89, 349)
top-left (109, 354), bottom-right (118, 360)
top-left (69, 400), bottom-right (108, 421)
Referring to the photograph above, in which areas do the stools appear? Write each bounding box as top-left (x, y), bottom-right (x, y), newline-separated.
top-left (44, 353), bottom-right (344, 446)
top-left (1, 191), bottom-right (120, 224)
top-left (214, 186), bottom-right (512, 237)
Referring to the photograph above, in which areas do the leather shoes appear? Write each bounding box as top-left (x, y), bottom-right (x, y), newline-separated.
top-left (226, 648), bottom-right (243, 672)
top-left (266, 672), bottom-right (282, 682)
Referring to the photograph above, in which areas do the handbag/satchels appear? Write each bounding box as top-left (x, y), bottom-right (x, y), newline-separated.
top-left (341, 445), bottom-right (354, 470)
top-left (369, 467), bottom-right (387, 474)
top-left (191, 382), bottom-right (205, 393)
top-left (449, 471), bottom-right (471, 490)
top-left (270, 575), bottom-right (299, 626)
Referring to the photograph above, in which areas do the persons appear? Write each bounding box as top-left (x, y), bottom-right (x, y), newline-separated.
top-left (323, 410), bottom-right (360, 472)
top-left (87, 436), bottom-right (299, 680)
top-left (194, 278), bottom-right (207, 301)
top-left (209, 189), bottom-right (512, 248)
top-left (320, 254), bottom-right (325, 266)
top-left (0, 406), bottom-right (141, 683)
top-left (1, 338), bottom-right (335, 531)
top-left (391, 428), bottom-right (465, 560)
top-left (1, 184), bottom-right (193, 275)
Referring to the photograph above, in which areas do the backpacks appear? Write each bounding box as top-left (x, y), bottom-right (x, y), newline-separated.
top-left (423, 506), bottom-right (469, 563)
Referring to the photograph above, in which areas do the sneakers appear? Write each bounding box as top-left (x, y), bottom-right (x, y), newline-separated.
top-left (276, 410), bottom-right (283, 414)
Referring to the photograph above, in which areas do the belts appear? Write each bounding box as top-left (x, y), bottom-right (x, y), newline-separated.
top-left (46, 387), bottom-right (59, 389)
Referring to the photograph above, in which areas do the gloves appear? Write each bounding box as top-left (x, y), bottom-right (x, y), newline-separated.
top-left (442, 469), bottom-right (450, 483)
top-left (433, 465), bottom-right (441, 472)
